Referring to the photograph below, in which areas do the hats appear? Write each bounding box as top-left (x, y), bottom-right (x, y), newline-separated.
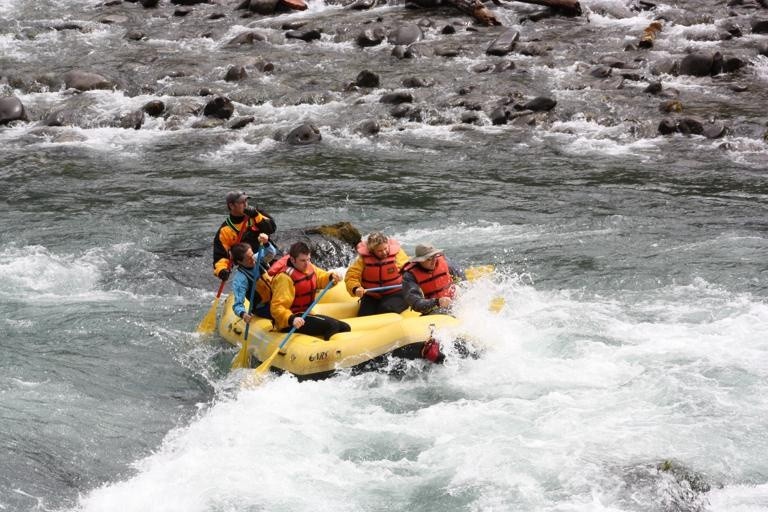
top-left (226, 191), bottom-right (251, 203)
top-left (411, 244), bottom-right (445, 263)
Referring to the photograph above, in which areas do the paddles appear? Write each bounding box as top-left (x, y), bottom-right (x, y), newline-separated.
top-left (363, 264), bottom-right (495, 292)
top-left (254, 278), bottom-right (335, 374)
top-left (232, 239), bottom-right (263, 369)
top-left (198, 215), bottom-right (249, 332)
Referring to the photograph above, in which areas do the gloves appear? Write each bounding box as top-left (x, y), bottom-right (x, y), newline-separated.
top-left (218, 270), bottom-right (232, 280)
top-left (243, 206), bottom-right (259, 218)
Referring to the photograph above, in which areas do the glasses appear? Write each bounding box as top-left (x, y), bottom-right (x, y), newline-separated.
top-left (428, 254), bottom-right (442, 261)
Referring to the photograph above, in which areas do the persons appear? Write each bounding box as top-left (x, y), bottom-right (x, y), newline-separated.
top-left (210, 190), bottom-right (277, 281)
top-left (268, 240), bottom-right (350, 340)
top-left (230, 232), bottom-right (280, 322)
top-left (397, 242), bottom-right (467, 318)
top-left (342, 231), bottom-right (415, 316)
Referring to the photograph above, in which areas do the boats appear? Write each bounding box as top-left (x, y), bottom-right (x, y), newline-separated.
top-left (217, 276), bottom-right (489, 384)
